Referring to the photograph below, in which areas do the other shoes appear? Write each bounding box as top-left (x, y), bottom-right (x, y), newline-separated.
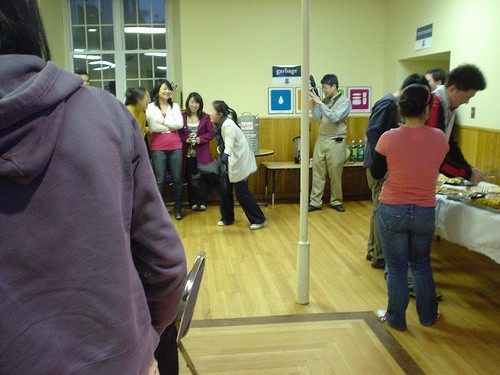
top-left (217, 220), bottom-right (225, 225)
top-left (409, 287), bottom-right (442, 299)
top-left (377, 309), bottom-right (388, 324)
top-left (367, 252), bottom-right (385, 269)
top-left (250, 219), bottom-right (267, 230)
top-left (174, 207), bottom-right (182, 220)
top-left (308, 204), bottom-right (321, 212)
top-left (191, 204), bottom-right (206, 211)
top-left (330, 204), bottom-right (345, 212)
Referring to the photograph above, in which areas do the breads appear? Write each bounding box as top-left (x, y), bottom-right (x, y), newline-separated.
top-left (435, 174), bottom-right (500, 209)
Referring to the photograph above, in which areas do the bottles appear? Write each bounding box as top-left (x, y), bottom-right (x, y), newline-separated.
top-left (357, 140), bottom-right (364, 161)
top-left (349, 140), bottom-right (357, 162)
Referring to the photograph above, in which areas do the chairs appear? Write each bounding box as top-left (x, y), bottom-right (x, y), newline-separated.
top-left (176, 252), bottom-right (205, 375)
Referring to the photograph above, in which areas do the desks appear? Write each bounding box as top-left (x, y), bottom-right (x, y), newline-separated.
top-left (260, 161), bottom-right (365, 205)
top-left (218, 149), bottom-right (274, 205)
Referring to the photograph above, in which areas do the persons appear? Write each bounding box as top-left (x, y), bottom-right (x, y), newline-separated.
top-left (383, 65), bottom-right (485, 302)
top-left (124, 86), bottom-right (149, 138)
top-left (76, 71), bottom-right (90, 85)
top-left (363, 74), bottom-right (427, 267)
top-left (424, 68), bottom-right (445, 90)
top-left (308, 75), bottom-right (351, 212)
top-left (145, 80), bottom-right (184, 219)
top-left (209, 100), bottom-right (267, 229)
top-left (179, 92), bottom-right (214, 211)
top-left (0, 0), bottom-right (187, 375)
top-left (370, 84), bottom-right (448, 328)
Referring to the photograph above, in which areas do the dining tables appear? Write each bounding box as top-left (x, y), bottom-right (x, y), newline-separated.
top-left (434, 181), bottom-right (500, 263)
